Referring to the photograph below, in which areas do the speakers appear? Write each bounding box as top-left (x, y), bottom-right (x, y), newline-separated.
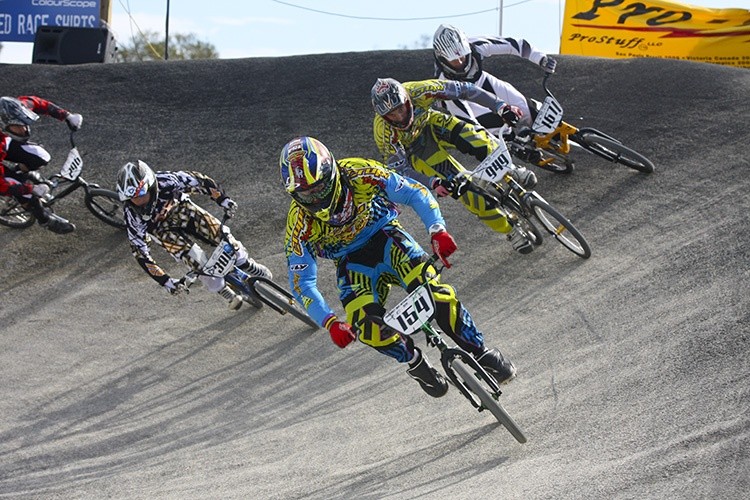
top-left (32, 25), bottom-right (118, 66)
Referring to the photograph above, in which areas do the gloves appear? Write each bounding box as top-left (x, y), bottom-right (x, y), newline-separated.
top-left (540, 57), bottom-right (556, 74)
top-left (498, 105), bottom-right (524, 125)
top-left (31, 184), bottom-right (51, 197)
top-left (330, 321), bottom-right (356, 348)
top-left (431, 230), bottom-right (457, 269)
top-left (221, 199), bottom-right (238, 213)
top-left (431, 177), bottom-right (455, 197)
top-left (66, 113), bottom-right (82, 131)
top-left (165, 278), bottom-right (187, 295)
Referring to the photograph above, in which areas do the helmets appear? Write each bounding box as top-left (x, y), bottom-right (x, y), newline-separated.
top-left (371, 78), bottom-right (414, 130)
top-left (0, 97), bottom-right (40, 141)
top-left (279, 137), bottom-right (341, 221)
top-left (433, 24), bottom-right (471, 74)
top-left (116, 160), bottom-right (158, 221)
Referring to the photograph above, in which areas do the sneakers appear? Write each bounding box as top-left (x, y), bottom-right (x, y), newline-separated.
top-left (40, 213), bottom-right (76, 233)
top-left (406, 346), bottom-right (449, 398)
top-left (474, 348), bottom-right (517, 384)
top-left (512, 165), bottom-right (537, 189)
top-left (508, 228), bottom-right (533, 254)
top-left (242, 258), bottom-right (272, 281)
top-left (217, 285), bottom-right (242, 309)
top-left (517, 148), bottom-right (544, 163)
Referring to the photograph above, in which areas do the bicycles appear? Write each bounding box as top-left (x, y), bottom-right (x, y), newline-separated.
top-left (0, 120), bottom-right (132, 230)
top-left (436, 115), bottom-right (592, 260)
top-left (334, 243), bottom-right (528, 446)
top-left (494, 56), bottom-right (656, 176)
top-left (169, 205), bottom-right (324, 331)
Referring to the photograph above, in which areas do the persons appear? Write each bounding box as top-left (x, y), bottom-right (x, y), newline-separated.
top-left (0, 95), bottom-right (84, 234)
top-left (279, 136), bottom-right (517, 399)
top-left (371, 24), bottom-right (558, 253)
top-left (116, 159), bottom-right (272, 310)
